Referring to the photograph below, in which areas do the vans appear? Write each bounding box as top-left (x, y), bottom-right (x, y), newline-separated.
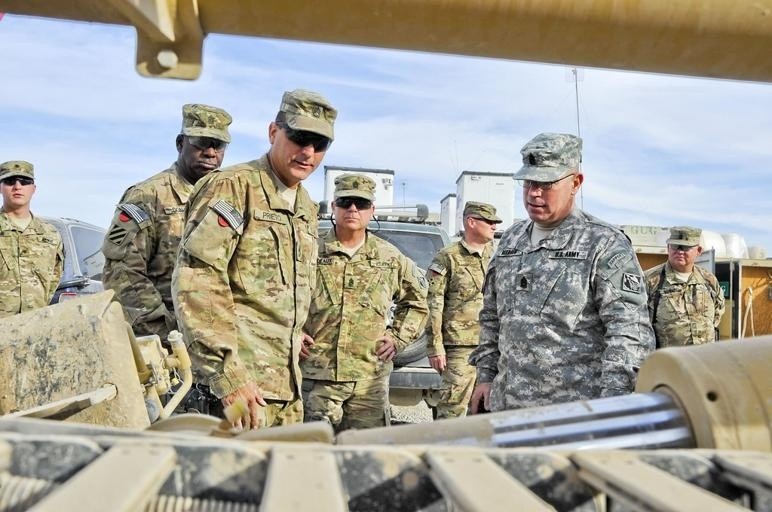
top-left (34, 216), bottom-right (108, 306)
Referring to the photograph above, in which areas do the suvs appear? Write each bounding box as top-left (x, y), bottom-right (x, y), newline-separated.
top-left (318, 199), bottom-right (452, 390)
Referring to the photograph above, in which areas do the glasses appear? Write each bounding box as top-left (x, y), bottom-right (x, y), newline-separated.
top-left (2, 178), bottom-right (33, 186)
top-left (517, 176), bottom-right (572, 191)
top-left (670, 245), bottom-right (692, 252)
top-left (188, 137), bottom-right (227, 150)
top-left (335, 197), bottom-right (372, 210)
top-left (280, 124), bottom-right (332, 152)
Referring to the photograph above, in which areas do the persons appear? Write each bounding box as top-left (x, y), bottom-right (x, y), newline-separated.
top-left (468, 132), bottom-right (656, 413)
top-left (169, 92), bottom-right (336, 433)
top-left (299, 174), bottom-right (428, 431)
top-left (427, 201), bottom-right (499, 418)
top-left (0, 156), bottom-right (65, 324)
top-left (102, 103), bottom-right (232, 353)
top-left (641, 227), bottom-right (725, 348)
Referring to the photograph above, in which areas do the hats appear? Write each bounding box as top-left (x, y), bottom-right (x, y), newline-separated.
top-left (333, 173), bottom-right (376, 203)
top-left (279, 88), bottom-right (337, 142)
top-left (181, 103), bottom-right (233, 144)
top-left (666, 225), bottom-right (702, 247)
top-left (0, 161), bottom-right (34, 182)
top-left (512, 132), bottom-right (583, 184)
top-left (463, 201), bottom-right (503, 225)
